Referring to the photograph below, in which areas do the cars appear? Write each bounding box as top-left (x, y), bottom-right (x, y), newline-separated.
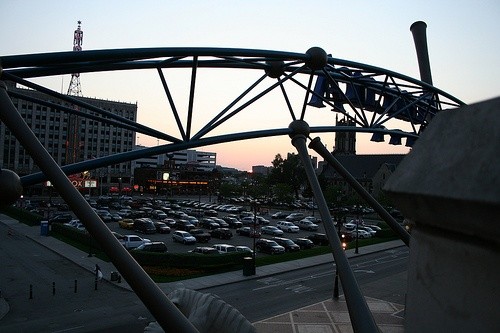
top-left (271, 210), bottom-right (292, 219)
top-left (261, 226), bottom-right (284, 236)
top-left (218, 194), bottom-right (400, 215)
top-left (331, 217), bottom-right (382, 242)
top-left (236, 227), bottom-right (261, 237)
top-left (25, 194), bottom-right (244, 252)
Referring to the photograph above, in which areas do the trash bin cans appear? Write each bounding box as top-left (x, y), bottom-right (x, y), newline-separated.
top-left (243, 256), bottom-right (254, 276)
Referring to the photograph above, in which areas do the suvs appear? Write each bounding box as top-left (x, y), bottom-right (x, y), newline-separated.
top-left (286, 212), bottom-right (304, 222)
top-left (188, 233), bottom-right (330, 256)
top-left (276, 217), bottom-right (322, 233)
top-left (172, 228), bottom-right (233, 245)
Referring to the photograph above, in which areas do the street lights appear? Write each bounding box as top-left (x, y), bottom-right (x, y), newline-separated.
top-left (353, 204), bottom-right (363, 254)
top-left (118, 178), bottom-right (122, 194)
top-left (47, 180), bottom-right (51, 226)
top-left (250, 199), bottom-right (261, 275)
top-left (19, 195), bottom-right (23, 223)
